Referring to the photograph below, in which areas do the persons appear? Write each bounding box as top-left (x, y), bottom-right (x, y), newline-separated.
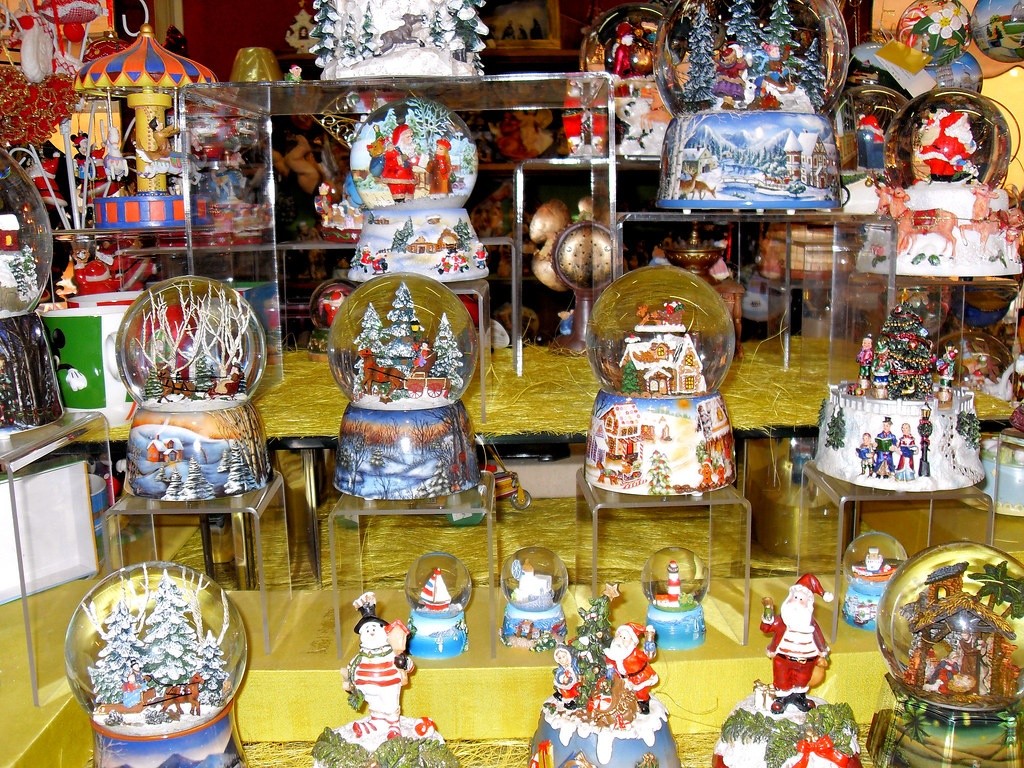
top-left (214, 363), bottom-right (242, 394)
top-left (381, 124), bottom-right (420, 201)
top-left (602, 623), bottom-right (660, 715)
top-left (412, 338), bottom-right (431, 366)
top-left (340, 593), bottom-right (416, 723)
top-left (856, 331), bottom-right (959, 400)
top-left (238, 132), bottom-right (326, 241)
top-left (21, 108), bottom-right (173, 294)
top-left (919, 111), bottom-right (979, 183)
top-left (425, 138), bottom-right (452, 194)
top-left (553, 643), bottom-right (581, 711)
top-left (925, 628), bottom-right (986, 693)
top-left (559, 21), bottom-right (809, 149)
top-left (760, 573), bottom-right (829, 715)
top-left (128, 659), bottom-right (150, 687)
